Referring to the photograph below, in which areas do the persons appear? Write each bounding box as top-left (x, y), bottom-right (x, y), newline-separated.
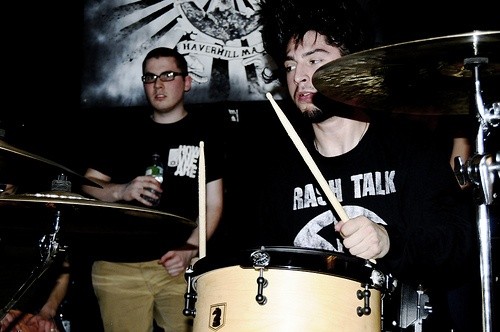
top-left (224, 23), bottom-right (424, 275)
top-left (0, 249), bottom-right (71, 332)
top-left (78, 46), bottom-right (223, 332)
top-left (448, 138), bottom-right (479, 188)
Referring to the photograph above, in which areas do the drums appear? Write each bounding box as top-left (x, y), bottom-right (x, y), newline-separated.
top-left (184, 244), bottom-right (394, 332)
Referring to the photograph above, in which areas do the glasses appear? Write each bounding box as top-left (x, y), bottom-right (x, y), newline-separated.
top-left (142, 70), bottom-right (183, 84)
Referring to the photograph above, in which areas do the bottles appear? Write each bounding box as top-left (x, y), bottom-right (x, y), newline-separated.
top-left (143, 154), bottom-right (164, 200)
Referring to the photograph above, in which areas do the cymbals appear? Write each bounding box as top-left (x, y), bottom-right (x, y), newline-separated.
top-left (0, 140), bottom-right (102, 188)
top-left (0, 190), bottom-right (197, 227)
top-left (311, 29), bottom-right (500, 115)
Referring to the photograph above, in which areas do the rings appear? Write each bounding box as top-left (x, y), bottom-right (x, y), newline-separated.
top-left (50, 328), bottom-right (55, 332)
